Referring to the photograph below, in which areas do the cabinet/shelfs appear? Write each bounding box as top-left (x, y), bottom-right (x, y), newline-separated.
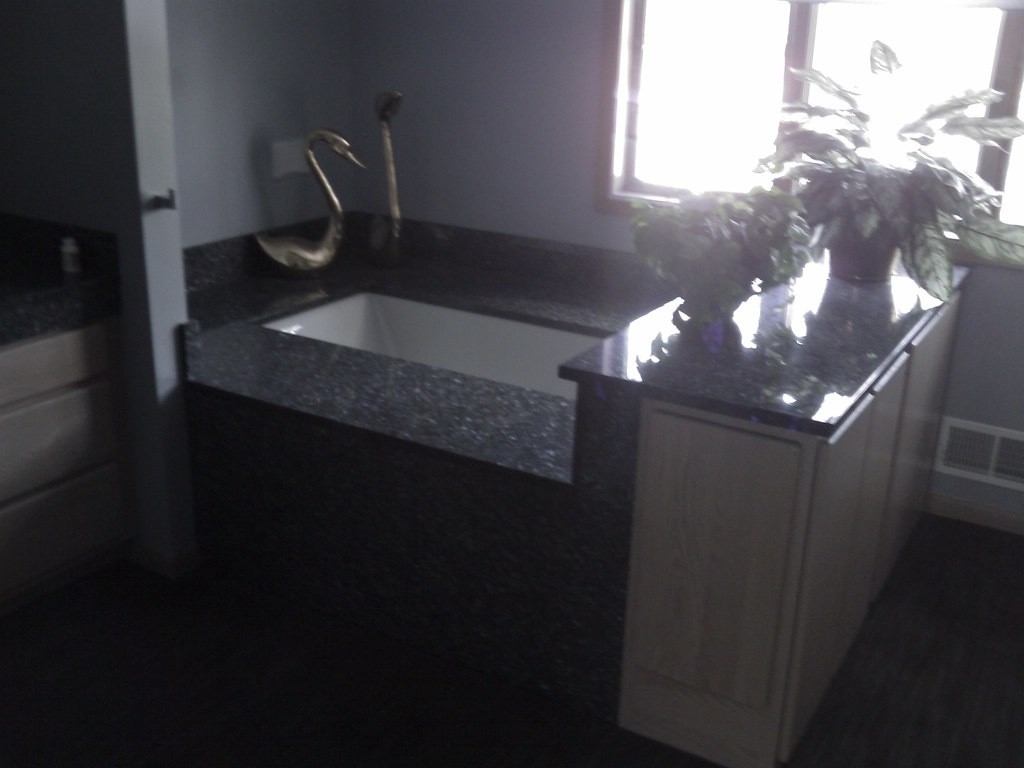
top-left (618, 291), bottom-right (969, 768)
top-left (0, 317), bottom-right (138, 619)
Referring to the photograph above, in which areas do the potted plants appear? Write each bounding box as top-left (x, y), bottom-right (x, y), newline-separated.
top-left (757, 41), bottom-right (1024, 304)
top-left (616, 187), bottom-right (811, 332)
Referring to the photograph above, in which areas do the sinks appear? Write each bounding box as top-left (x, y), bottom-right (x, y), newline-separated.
top-left (260, 285), bottom-right (611, 402)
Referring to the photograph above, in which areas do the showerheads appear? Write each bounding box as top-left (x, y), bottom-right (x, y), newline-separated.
top-left (370, 88), bottom-right (407, 241)
